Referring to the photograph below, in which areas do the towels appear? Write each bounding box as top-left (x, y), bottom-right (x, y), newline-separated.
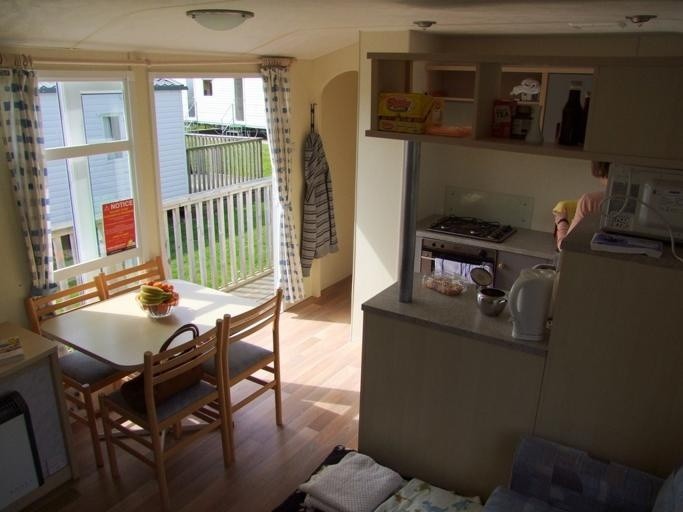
top-left (299, 452), bottom-right (402, 512)
top-left (375, 478), bottom-right (482, 512)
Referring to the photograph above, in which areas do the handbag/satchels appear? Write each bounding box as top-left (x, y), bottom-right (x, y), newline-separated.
top-left (121, 323), bottom-right (204, 415)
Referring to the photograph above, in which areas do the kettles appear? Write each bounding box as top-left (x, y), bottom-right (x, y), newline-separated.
top-left (506, 266), bottom-right (557, 341)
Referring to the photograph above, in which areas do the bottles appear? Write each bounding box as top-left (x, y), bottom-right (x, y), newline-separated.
top-left (558, 78), bottom-right (591, 148)
top-left (526, 106), bottom-right (544, 144)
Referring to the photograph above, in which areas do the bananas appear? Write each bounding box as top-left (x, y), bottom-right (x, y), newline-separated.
top-left (138, 285), bottom-right (171, 306)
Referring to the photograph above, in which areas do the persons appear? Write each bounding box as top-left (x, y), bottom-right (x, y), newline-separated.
top-left (551, 159), bottom-right (612, 255)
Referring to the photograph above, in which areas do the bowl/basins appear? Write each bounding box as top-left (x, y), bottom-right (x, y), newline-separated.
top-left (139, 302), bottom-right (175, 319)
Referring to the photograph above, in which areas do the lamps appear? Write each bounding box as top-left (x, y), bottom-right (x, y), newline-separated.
top-left (187, 9), bottom-right (254, 31)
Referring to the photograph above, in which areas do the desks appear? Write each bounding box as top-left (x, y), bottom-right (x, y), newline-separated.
top-left (0, 322), bottom-right (81, 512)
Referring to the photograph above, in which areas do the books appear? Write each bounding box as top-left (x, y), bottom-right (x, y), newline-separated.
top-left (0, 336), bottom-right (23, 360)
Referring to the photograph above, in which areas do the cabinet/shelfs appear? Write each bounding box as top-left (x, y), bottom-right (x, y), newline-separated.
top-left (365, 28), bottom-right (683, 170)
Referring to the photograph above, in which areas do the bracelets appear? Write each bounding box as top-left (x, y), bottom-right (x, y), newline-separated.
top-left (556, 217), bottom-right (569, 226)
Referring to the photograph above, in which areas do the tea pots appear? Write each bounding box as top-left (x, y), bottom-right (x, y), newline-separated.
top-left (469, 267), bottom-right (507, 317)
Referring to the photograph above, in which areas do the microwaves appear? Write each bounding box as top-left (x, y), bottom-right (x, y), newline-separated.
top-left (601, 162), bottom-right (683, 241)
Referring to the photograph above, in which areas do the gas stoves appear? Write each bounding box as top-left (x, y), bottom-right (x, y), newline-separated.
top-left (422, 215), bottom-right (518, 259)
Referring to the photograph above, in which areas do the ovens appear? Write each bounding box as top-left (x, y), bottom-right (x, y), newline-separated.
top-left (420, 251), bottom-right (497, 289)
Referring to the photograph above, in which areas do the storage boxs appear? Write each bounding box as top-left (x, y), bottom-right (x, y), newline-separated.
top-left (377, 91), bottom-right (433, 133)
